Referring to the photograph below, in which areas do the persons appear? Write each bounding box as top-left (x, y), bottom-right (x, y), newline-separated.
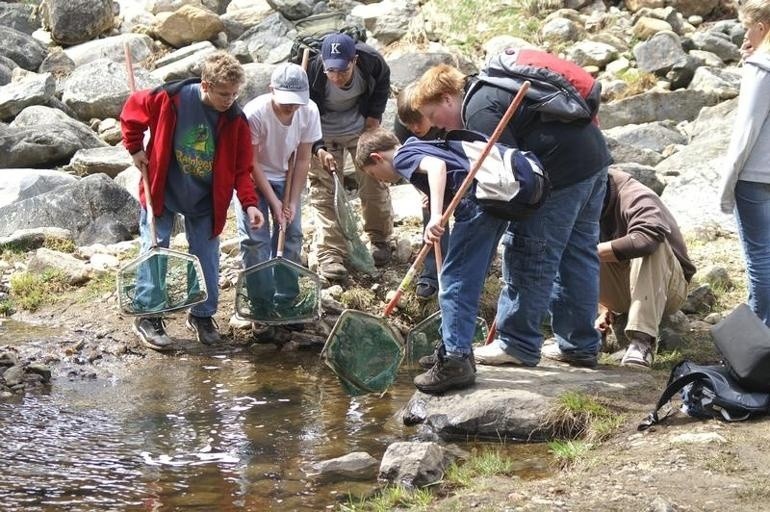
top-left (232, 60), bottom-right (325, 346)
top-left (118, 53), bottom-right (267, 354)
top-left (715, 2), bottom-right (769, 332)
top-left (304, 31), bottom-right (393, 283)
top-left (353, 126), bottom-right (512, 397)
top-left (595, 166), bottom-right (697, 372)
top-left (408, 63), bottom-right (617, 370)
top-left (392, 79), bottom-right (450, 301)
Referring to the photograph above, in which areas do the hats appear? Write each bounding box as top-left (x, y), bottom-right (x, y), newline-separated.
top-left (322, 33), bottom-right (355, 70)
top-left (271, 62), bottom-right (310, 105)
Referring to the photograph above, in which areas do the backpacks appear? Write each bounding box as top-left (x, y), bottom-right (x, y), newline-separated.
top-left (396, 130), bottom-right (552, 219)
top-left (710, 304), bottom-right (770, 387)
top-left (638, 359), bottom-right (770, 431)
top-left (460, 49), bottom-right (601, 128)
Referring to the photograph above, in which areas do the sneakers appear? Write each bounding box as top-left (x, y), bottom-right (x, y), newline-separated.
top-left (414, 339), bottom-right (523, 392)
top-left (370, 240), bottom-right (392, 266)
top-left (252, 308), bottom-right (304, 341)
top-left (132, 316), bottom-right (174, 350)
top-left (621, 339), bottom-right (653, 369)
top-left (541, 342), bottom-right (596, 366)
top-left (322, 262), bottom-right (348, 280)
top-left (186, 313), bottom-right (221, 344)
top-left (415, 283), bottom-right (437, 299)
